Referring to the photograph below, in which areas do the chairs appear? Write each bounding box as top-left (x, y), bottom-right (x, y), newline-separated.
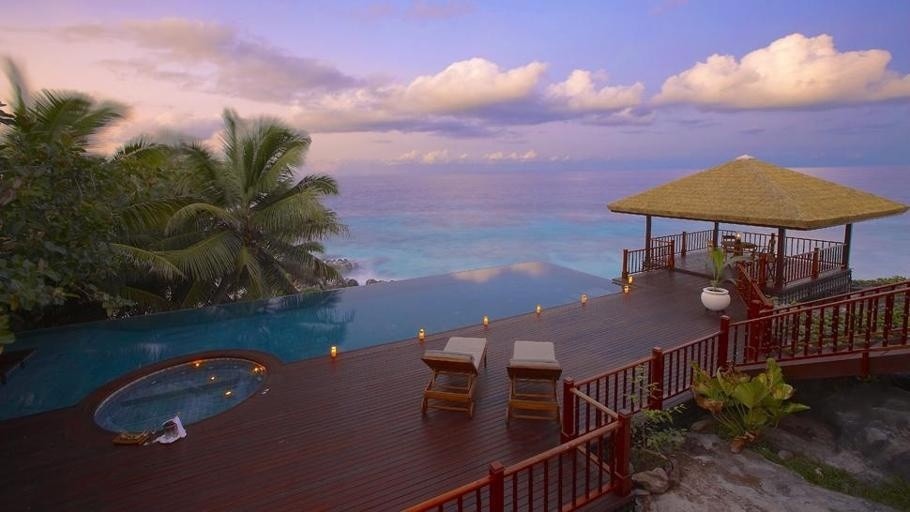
top-left (417, 336), bottom-right (562, 427)
top-left (707, 234), bottom-right (774, 280)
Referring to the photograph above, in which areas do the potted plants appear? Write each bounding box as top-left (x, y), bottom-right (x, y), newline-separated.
top-left (699, 238), bottom-right (754, 317)
